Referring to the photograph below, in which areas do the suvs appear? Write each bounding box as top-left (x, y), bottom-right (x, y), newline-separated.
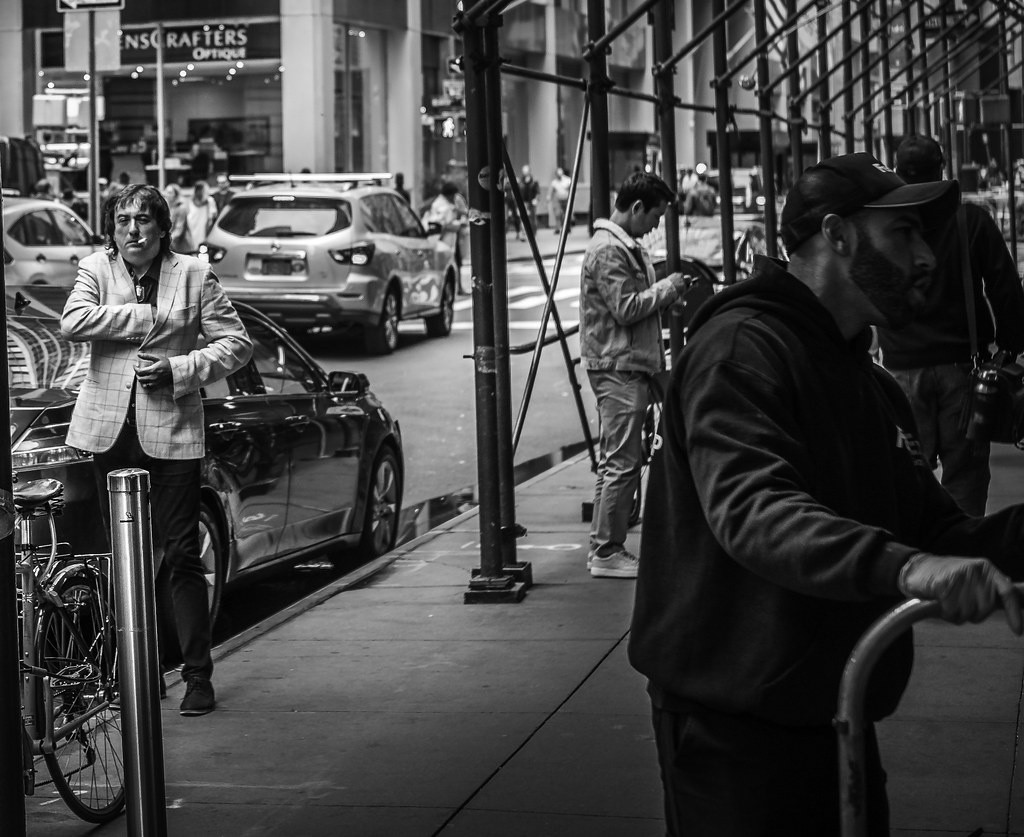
top-left (196, 182), bottom-right (458, 356)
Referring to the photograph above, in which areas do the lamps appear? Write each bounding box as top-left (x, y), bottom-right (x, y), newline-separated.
top-left (737, 70), bottom-right (758, 89)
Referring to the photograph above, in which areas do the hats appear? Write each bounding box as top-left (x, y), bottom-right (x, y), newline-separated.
top-left (896, 135), bottom-right (947, 177)
top-left (780, 151), bottom-right (961, 256)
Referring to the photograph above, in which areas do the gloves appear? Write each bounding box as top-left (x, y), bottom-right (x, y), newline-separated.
top-left (899, 553), bottom-right (1022, 636)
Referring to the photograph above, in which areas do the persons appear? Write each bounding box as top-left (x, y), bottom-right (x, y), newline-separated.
top-left (629, 150), bottom-right (1024, 837)
top-left (579, 173), bottom-right (691, 579)
top-left (865, 134), bottom-right (1023, 518)
top-left (33, 161), bottom-right (724, 295)
top-left (59, 184), bottom-right (254, 716)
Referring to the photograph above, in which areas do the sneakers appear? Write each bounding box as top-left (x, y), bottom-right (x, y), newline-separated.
top-left (590, 552), bottom-right (640, 580)
top-left (177, 678), bottom-right (216, 717)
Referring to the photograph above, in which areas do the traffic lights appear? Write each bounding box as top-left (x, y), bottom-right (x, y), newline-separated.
top-left (435, 116), bottom-right (466, 139)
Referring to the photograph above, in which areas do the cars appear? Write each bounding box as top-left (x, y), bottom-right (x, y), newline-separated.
top-left (3, 284), bottom-right (406, 637)
top-left (1, 195), bottom-right (108, 285)
top-left (706, 168), bottom-right (778, 210)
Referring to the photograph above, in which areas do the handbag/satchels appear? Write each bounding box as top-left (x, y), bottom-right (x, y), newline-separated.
top-left (971, 353), bottom-right (1024, 445)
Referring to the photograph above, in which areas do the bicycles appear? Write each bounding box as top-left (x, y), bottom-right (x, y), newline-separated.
top-left (11, 478), bottom-right (130, 823)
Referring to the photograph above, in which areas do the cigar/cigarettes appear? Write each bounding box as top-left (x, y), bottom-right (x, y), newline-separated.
top-left (136, 237), bottom-right (149, 244)
